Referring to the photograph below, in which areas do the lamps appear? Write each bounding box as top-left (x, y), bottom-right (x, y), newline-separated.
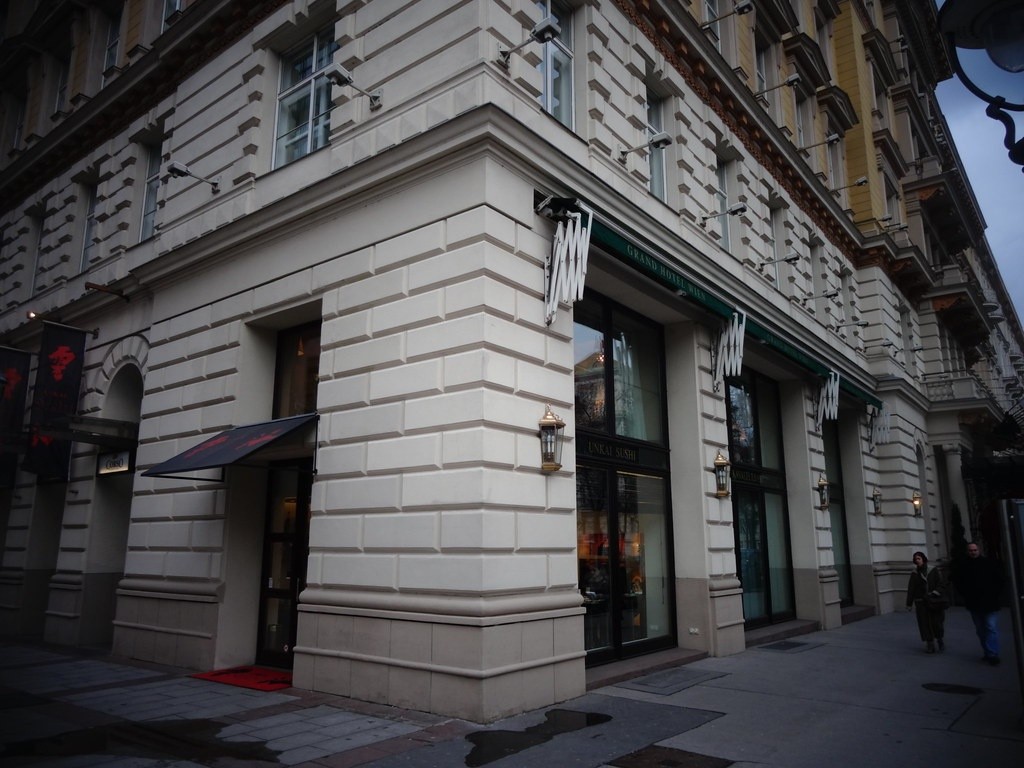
top-left (758, 254), bottom-right (800, 268)
top-left (873, 489), bottom-right (883, 515)
top-left (539, 413), bottom-right (564, 472)
top-left (167, 163), bottom-right (223, 193)
top-left (912, 492), bottom-right (922, 515)
top-left (25, 311), bottom-right (100, 339)
top-left (802, 290), bottom-right (839, 304)
top-left (713, 454), bottom-right (730, 496)
top-left (699, 202), bottom-right (748, 227)
top-left (616, 132), bottom-right (671, 162)
top-left (325, 64), bottom-right (384, 110)
top-left (862, 340), bottom-right (894, 352)
top-left (497, 18), bottom-right (561, 66)
top-left (819, 474), bottom-right (829, 510)
top-left (834, 320), bottom-right (870, 331)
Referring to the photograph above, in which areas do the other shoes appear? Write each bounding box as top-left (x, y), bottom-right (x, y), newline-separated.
top-left (988, 656), bottom-right (1001, 666)
top-left (928, 648), bottom-right (935, 653)
top-left (937, 639), bottom-right (945, 652)
top-left (980, 654), bottom-right (989, 661)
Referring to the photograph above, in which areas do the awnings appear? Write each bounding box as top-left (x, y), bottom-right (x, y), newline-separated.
top-left (140, 409), bottom-right (320, 483)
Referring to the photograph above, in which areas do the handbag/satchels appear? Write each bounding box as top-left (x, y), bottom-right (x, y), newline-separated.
top-left (923, 592), bottom-right (949, 610)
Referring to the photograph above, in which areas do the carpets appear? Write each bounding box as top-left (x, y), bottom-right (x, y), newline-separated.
top-left (191, 665), bottom-right (294, 693)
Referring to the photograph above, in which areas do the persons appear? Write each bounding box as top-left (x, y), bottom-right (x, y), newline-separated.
top-left (904, 552), bottom-right (950, 652)
top-left (952, 542), bottom-right (1008, 666)
